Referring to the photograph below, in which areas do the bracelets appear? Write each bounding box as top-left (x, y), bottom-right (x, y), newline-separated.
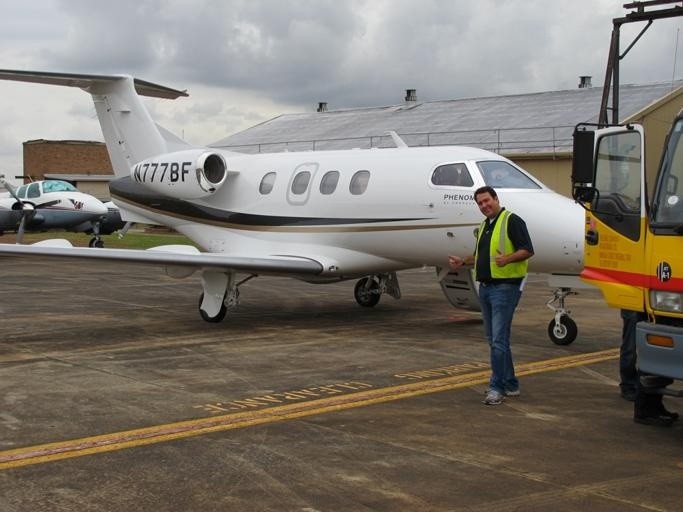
top-left (460, 258), bottom-right (466, 266)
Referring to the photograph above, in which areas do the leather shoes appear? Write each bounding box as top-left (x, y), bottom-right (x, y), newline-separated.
top-left (621, 388), bottom-right (638, 401)
top-left (633, 414), bottom-right (673, 427)
top-left (661, 408), bottom-right (678, 420)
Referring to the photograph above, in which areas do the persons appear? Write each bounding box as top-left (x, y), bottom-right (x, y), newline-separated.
top-left (620, 309), bottom-right (679, 428)
top-left (448, 185), bottom-right (535, 406)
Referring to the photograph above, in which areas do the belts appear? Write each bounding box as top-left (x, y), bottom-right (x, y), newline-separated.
top-left (481, 281), bottom-right (519, 289)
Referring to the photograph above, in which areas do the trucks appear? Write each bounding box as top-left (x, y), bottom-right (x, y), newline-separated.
top-left (570, 108), bottom-right (683, 434)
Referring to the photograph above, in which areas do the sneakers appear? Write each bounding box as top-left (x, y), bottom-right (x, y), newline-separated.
top-left (483, 387), bottom-right (520, 397)
top-left (481, 390), bottom-right (507, 405)
